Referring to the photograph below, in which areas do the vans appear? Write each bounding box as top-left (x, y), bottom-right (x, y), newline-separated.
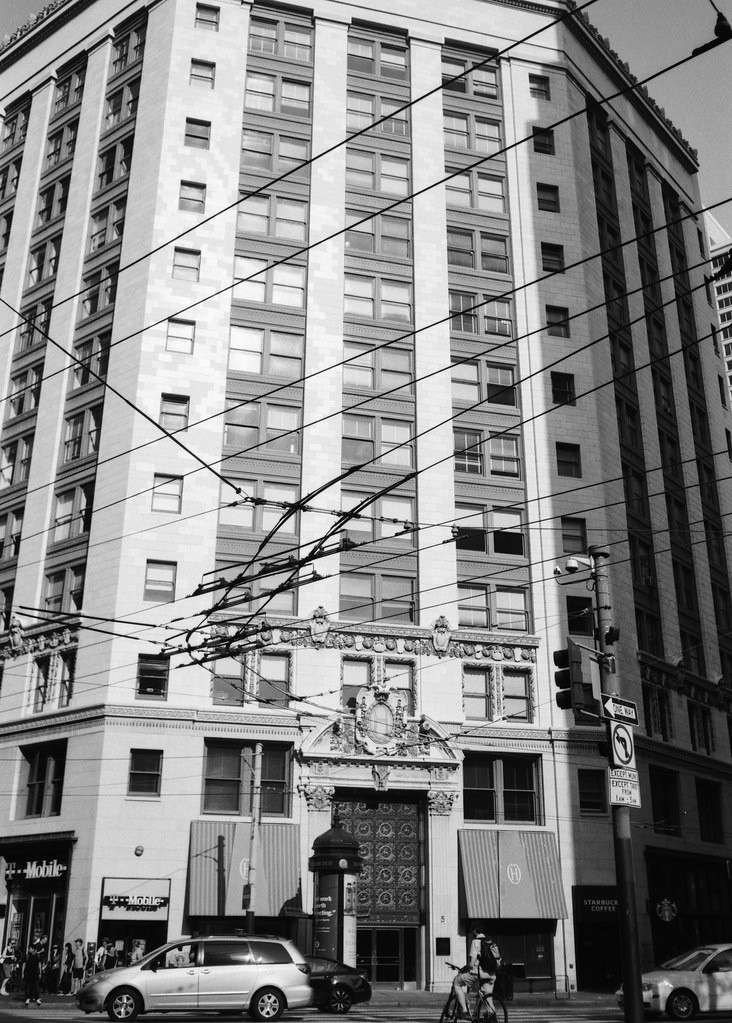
top-left (76, 933), bottom-right (314, 1022)
top-left (615, 943), bottom-right (732, 1021)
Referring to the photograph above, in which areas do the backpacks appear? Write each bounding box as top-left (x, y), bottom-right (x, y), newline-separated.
top-left (475, 936), bottom-right (502, 976)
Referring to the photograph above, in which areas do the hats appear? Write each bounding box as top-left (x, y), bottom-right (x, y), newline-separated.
top-left (50, 945), bottom-right (60, 952)
top-left (75, 939), bottom-right (83, 944)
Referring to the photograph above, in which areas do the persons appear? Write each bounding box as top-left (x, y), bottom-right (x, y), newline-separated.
top-left (169, 946), bottom-right (189, 968)
top-left (0, 936), bottom-right (143, 1005)
top-left (454, 926), bottom-right (502, 1021)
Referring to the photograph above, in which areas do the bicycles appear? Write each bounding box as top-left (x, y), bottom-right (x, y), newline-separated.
top-left (439, 962), bottom-right (509, 1023)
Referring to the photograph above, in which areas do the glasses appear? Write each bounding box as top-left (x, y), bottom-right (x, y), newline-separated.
top-left (10, 943), bottom-right (15, 945)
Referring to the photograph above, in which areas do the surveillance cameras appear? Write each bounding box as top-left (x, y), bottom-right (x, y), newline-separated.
top-left (565, 559), bottom-right (578, 574)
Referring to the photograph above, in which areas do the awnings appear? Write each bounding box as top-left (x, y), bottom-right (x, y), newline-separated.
top-left (189, 820), bottom-right (302, 918)
top-left (457, 829), bottom-right (569, 920)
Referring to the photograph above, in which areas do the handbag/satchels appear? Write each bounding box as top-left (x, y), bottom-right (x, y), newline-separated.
top-left (0, 955), bottom-right (5, 964)
top-left (59, 967), bottom-right (72, 994)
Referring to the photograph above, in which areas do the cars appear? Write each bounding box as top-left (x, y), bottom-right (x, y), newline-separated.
top-left (303, 955), bottom-right (373, 1014)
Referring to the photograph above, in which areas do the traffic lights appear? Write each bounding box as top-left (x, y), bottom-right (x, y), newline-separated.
top-left (554, 637), bottom-right (584, 711)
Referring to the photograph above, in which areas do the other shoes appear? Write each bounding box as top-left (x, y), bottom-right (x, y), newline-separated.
top-left (35, 999), bottom-right (41, 1004)
top-left (25, 999), bottom-right (31, 1005)
top-left (0, 988), bottom-right (9, 996)
top-left (65, 993), bottom-right (72, 996)
top-left (453, 1010), bottom-right (470, 1020)
top-left (57, 992), bottom-right (64, 997)
